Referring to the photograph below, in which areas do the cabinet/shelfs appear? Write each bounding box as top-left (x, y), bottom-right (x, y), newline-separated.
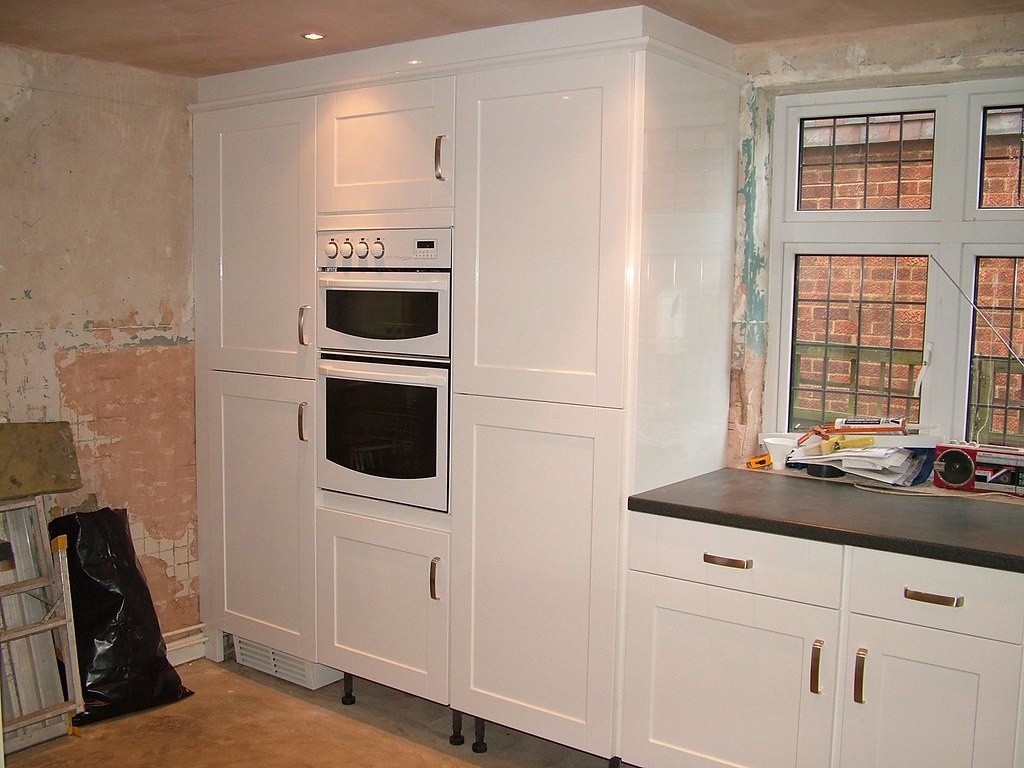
top-left (618, 508), bottom-right (1023, 767)
top-left (186, 96), bottom-right (317, 665)
top-left (318, 76), bottom-right (464, 212)
top-left (317, 506), bottom-right (448, 706)
top-left (459, 51), bottom-right (619, 759)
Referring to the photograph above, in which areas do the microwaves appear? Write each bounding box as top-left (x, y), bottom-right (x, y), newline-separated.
top-left (312, 227), bottom-right (452, 361)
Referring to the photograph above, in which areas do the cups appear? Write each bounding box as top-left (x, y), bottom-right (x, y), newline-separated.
top-left (763, 437), bottom-right (796, 470)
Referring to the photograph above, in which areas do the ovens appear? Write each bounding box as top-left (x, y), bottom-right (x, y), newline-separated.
top-left (314, 358), bottom-right (450, 514)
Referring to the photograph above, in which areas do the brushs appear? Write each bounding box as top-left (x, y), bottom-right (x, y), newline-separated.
top-left (803, 435), bottom-right (875, 456)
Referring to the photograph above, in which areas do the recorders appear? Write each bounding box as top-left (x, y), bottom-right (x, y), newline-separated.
top-left (934, 440), bottom-right (1024, 496)
